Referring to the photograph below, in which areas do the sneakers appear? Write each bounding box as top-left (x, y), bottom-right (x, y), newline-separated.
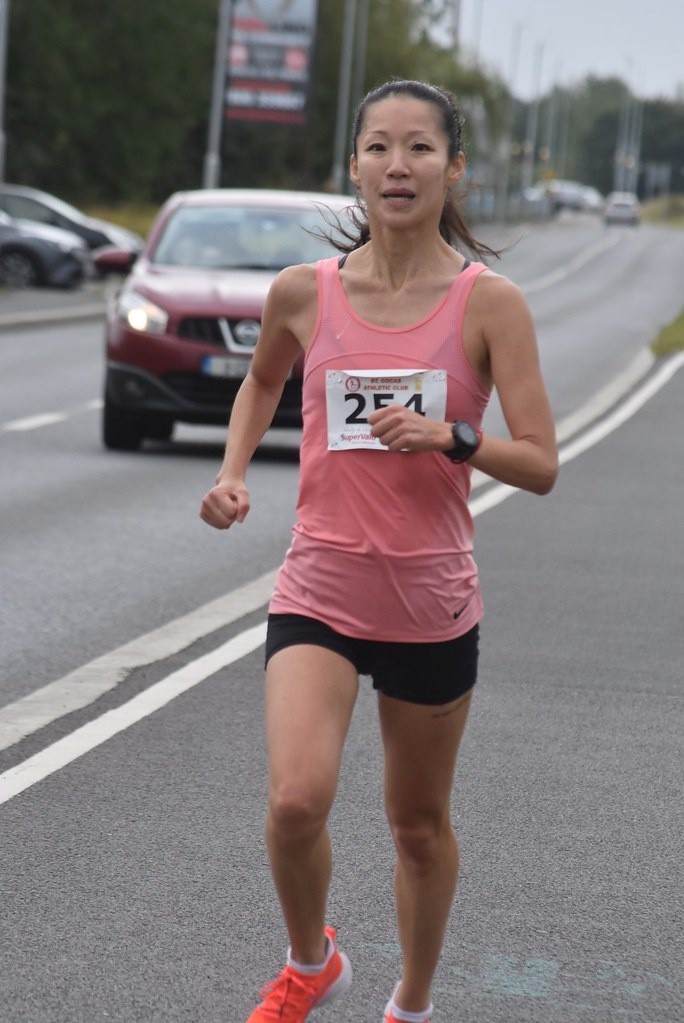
top-left (246, 925), bottom-right (352, 1023)
top-left (383, 994), bottom-right (429, 1023)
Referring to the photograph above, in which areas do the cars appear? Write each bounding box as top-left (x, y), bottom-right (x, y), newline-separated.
top-left (0, 207), bottom-right (93, 292)
top-left (535, 174), bottom-right (643, 230)
top-left (0, 179), bottom-right (146, 277)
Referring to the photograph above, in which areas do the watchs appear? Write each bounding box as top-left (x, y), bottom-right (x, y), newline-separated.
top-left (443, 420), bottom-right (479, 462)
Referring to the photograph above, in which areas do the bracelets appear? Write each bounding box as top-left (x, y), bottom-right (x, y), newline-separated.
top-left (453, 430), bottom-right (482, 464)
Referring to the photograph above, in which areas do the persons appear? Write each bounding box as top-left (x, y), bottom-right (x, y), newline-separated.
top-left (199, 80), bottom-right (559, 1023)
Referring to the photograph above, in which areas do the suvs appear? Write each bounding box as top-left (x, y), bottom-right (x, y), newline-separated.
top-left (88, 184), bottom-right (372, 450)
